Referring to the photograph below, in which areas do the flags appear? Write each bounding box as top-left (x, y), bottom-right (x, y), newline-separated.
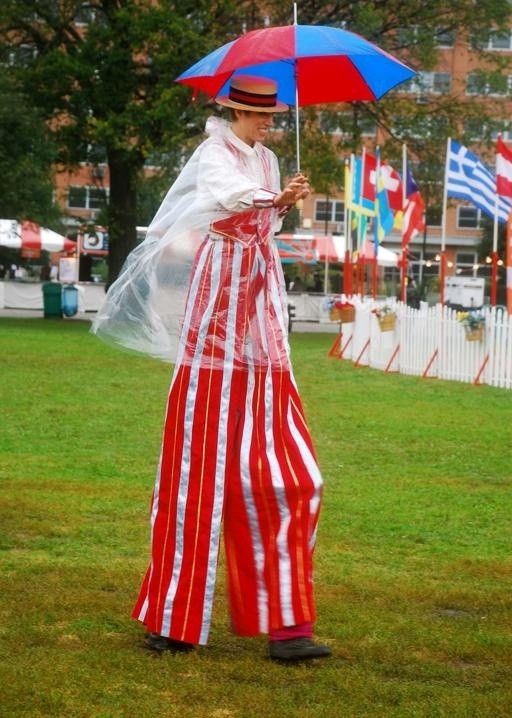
top-left (343, 152), bottom-right (428, 263)
top-left (447, 139), bottom-right (512, 223)
top-left (495, 138), bottom-right (512, 197)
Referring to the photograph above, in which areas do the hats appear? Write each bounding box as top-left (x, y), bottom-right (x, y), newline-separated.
top-left (215, 75), bottom-right (290, 113)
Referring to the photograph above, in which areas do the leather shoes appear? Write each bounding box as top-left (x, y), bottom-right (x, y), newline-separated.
top-left (269, 637), bottom-right (332, 659)
top-left (145, 632), bottom-right (194, 651)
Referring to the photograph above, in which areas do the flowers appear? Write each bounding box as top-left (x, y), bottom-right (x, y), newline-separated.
top-left (456, 310), bottom-right (484, 331)
top-left (322, 294), bottom-right (354, 310)
top-left (370, 305), bottom-right (391, 317)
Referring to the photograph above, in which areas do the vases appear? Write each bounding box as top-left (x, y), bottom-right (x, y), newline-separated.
top-left (464, 324), bottom-right (484, 341)
top-left (379, 311), bottom-right (396, 332)
top-left (329, 309), bottom-right (354, 322)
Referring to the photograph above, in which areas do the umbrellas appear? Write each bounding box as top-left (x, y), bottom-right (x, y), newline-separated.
top-left (174, 0), bottom-right (421, 172)
top-left (0, 219), bottom-right (77, 253)
top-left (330, 233), bottom-right (400, 268)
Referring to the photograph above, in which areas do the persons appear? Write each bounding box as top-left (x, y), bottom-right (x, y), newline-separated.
top-left (406, 276), bottom-right (416, 293)
top-left (362, 273), bottom-right (370, 289)
top-left (130, 73), bottom-right (333, 662)
top-left (284, 271), bottom-right (342, 293)
top-left (0, 261), bottom-right (51, 281)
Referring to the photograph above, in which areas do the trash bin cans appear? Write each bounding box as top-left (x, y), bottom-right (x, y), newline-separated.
top-left (43, 283), bottom-right (63, 319)
top-left (63, 287), bottom-right (78, 317)
